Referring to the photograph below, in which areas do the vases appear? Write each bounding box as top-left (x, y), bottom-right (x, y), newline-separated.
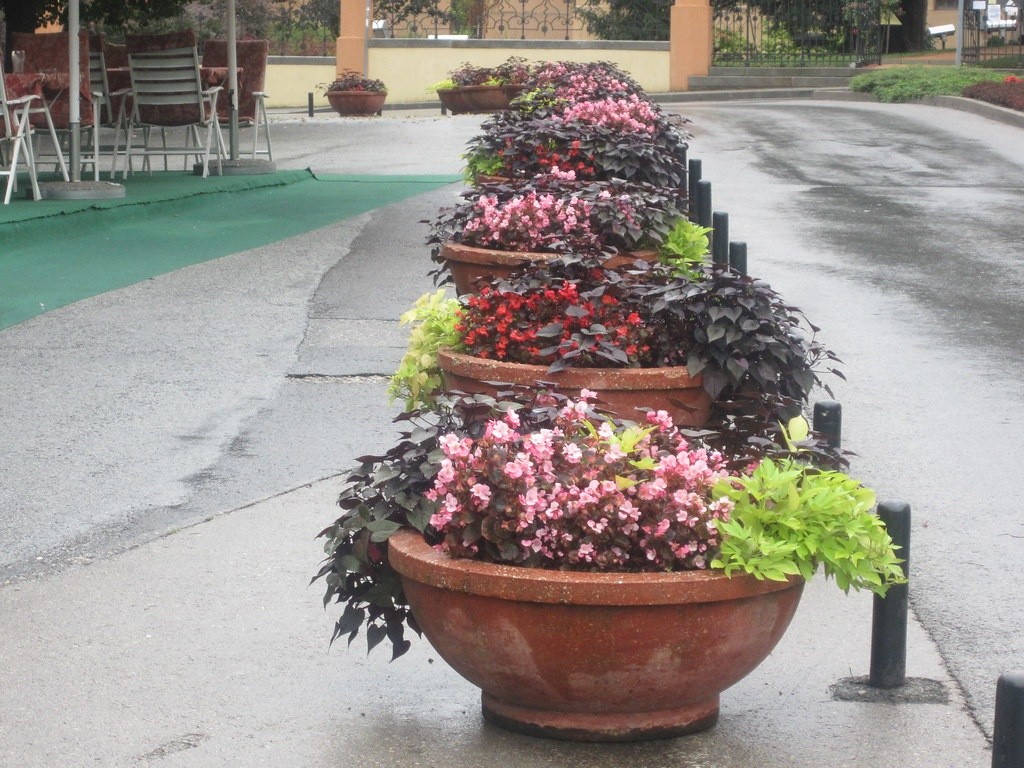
top-left (325, 91), bottom-right (387, 117)
top-left (436, 88), bottom-right (470, 116)
top-left (471, 85), bottom-right (512, 113)
top-left (460, 85), bottom-right (485, 113)
top-left (503, 83), bottom-right (524, 99)
top-left (387, 526), bottom-right (817, 745)
top-left (438, 347), bottom-right (715, 428)
top-left (438, 240), bottom-right (661, 301)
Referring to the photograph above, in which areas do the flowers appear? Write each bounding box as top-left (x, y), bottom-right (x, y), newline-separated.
top-left (417, 50), bottom-right (718, 287)
top-left (307, 376), bottom-right (910, 667)
top-left (386, 240), bottom-right (846, 446)
top-left (314, 66), bottom-right (389, 96)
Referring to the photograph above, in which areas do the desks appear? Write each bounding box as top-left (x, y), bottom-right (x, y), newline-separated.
top-left (104, 65), bottom-right (245, 177)
top-left (4, 72), bottom-right (69, 102)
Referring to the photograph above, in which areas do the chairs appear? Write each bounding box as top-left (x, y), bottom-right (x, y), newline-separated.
top-left (0, 29), bottom-right (272, 205)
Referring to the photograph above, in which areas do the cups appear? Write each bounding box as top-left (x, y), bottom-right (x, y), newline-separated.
top-left (12, 50), bottom-right (25, 74)
